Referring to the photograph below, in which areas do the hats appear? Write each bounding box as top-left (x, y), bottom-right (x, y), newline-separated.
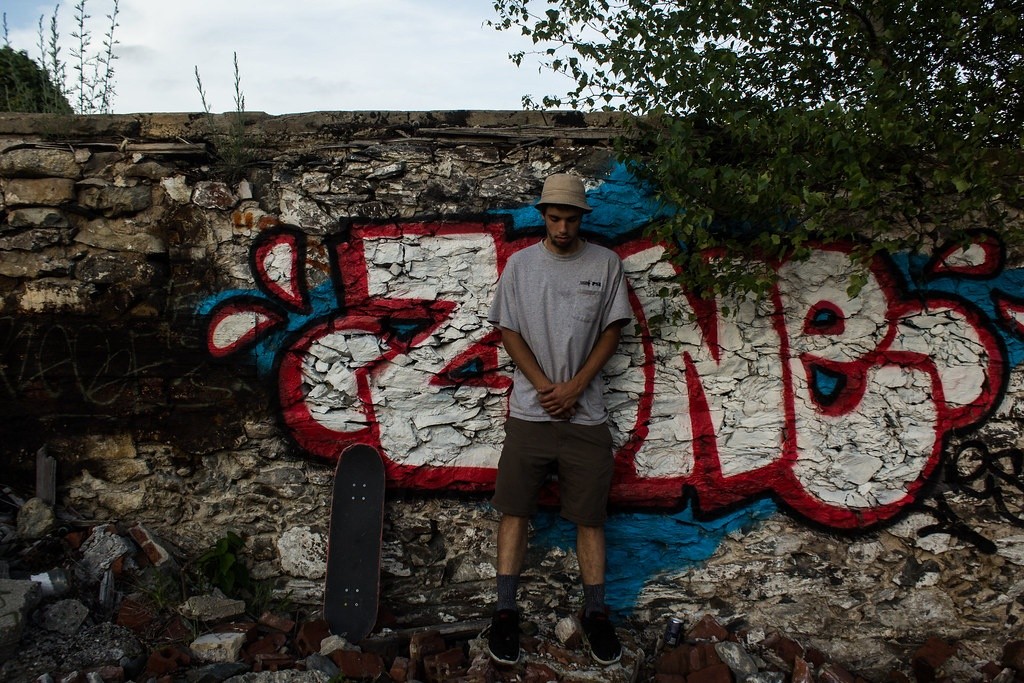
top-left (534, 173), bottom-right (593, 214)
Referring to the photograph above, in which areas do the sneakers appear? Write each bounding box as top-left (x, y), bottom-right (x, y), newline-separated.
top-left (481, 608), bottom-right (522, 664)
top-left (577, 603), bottom-right (621, 664)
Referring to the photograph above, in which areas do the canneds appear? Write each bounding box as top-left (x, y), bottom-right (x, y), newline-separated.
top-left (663, 618), bottom-right (685, 649)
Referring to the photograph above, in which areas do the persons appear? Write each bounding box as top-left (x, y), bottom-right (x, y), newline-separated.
top-left (484, 173), bottom-right (632, 666)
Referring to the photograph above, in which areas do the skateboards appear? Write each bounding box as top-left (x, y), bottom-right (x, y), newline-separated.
top-left (323, 444), bottom-right (385, 643)
